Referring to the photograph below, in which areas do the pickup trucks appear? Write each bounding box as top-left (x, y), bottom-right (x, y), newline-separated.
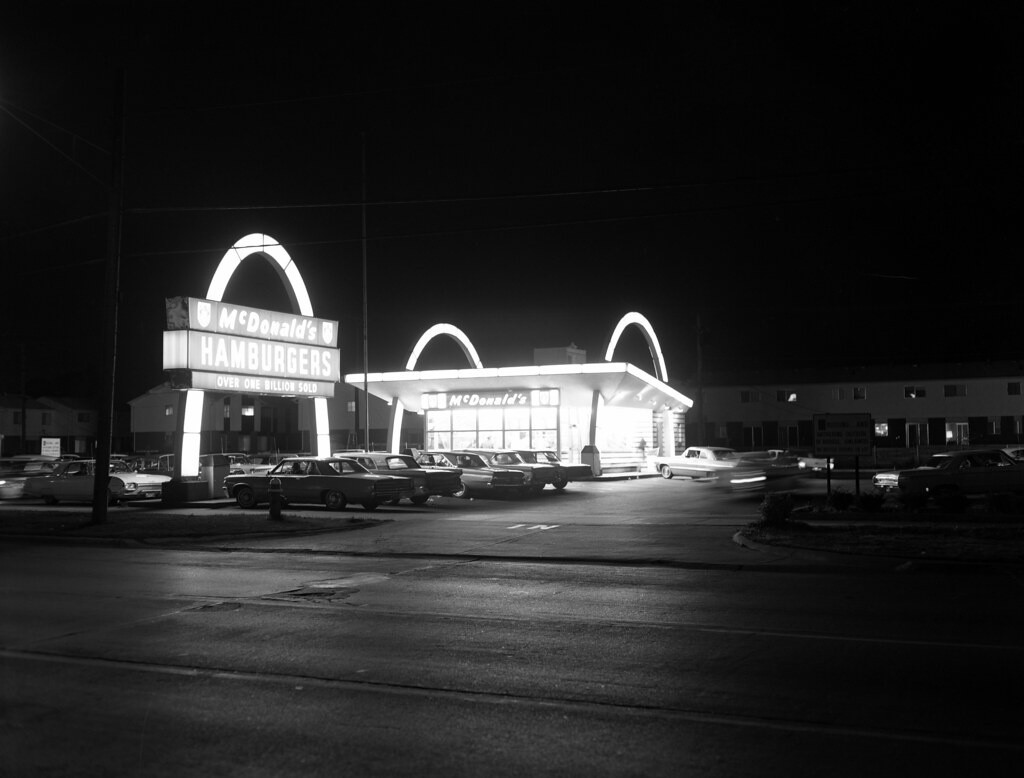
top-left (660, 446), bottom-right (747, 478)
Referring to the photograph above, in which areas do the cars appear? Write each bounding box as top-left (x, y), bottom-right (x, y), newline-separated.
top-left (1, 453), bottom-right (304, 508)
top-left (225, 449), bottom-right (413, 510)
top-left (409, 452), bottom-right (526, 496)
top-left (344, 453), bottom-right (465, 505)
top-left (480, 452), bottom-right (594, 496)
top-left (871, 449), bottom-right (1024, 494)
